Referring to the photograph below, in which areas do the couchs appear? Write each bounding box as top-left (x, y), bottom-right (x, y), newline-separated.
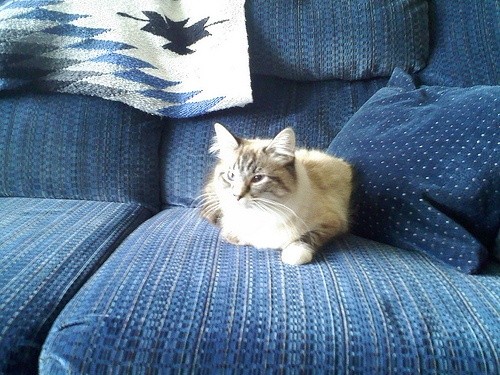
top-left (0, 0), bottom-right (500, 375)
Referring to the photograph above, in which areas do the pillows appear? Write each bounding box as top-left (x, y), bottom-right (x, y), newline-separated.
top-left (325, 68), bottom-right (500, 274)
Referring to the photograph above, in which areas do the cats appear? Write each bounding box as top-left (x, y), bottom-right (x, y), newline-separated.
top-left (190, 122), bottom-right (357, 266)
